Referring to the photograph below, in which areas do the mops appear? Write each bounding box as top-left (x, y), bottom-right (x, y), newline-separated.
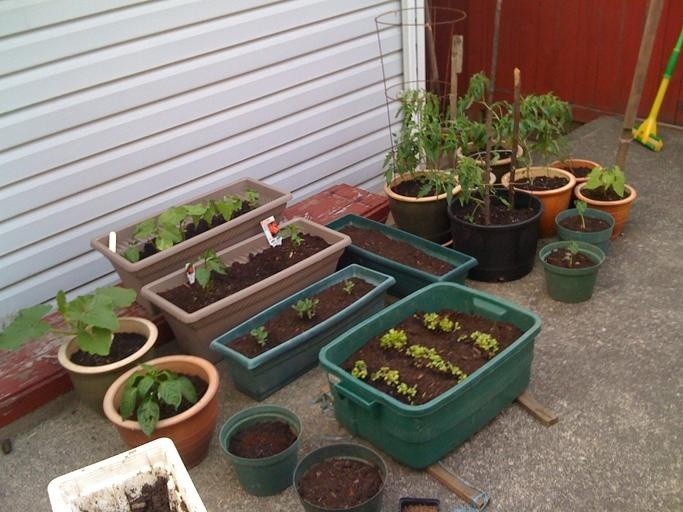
top-left (631, 26), bottom-right (683, 152)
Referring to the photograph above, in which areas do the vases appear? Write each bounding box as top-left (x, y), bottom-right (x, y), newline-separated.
top-left (325, 211), bottom-right (478, 298)
top-left (218, 404), bottom-right (303, 498)
top-left (47, 437), bottom-right (207, 512)
top-left (294, 444), bottom-right (388, 511)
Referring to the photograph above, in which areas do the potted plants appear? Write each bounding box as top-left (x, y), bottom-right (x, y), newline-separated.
top-left (209, 262), bottom-right (395, 401)
top-left (91, 177), bottom-right (293, 314)
top-left (383, 71), bottom-right (576, 282)
top-left (539, 239), bottom-right (606, 300)
top-left (317, 281), bottom-right (541, 471)
top-left (102, 353), bottom-right (219, 470)
top-left (553, 200), bottom-right (615, 256)
top-left (546, 157), bottom-right (602, 200)
top-left (1, 285), bottom-right (160, 418)
top-left (575, 165), bottom-right (636, 240)
top-left (139, 215), bottom-right (350, 356)
